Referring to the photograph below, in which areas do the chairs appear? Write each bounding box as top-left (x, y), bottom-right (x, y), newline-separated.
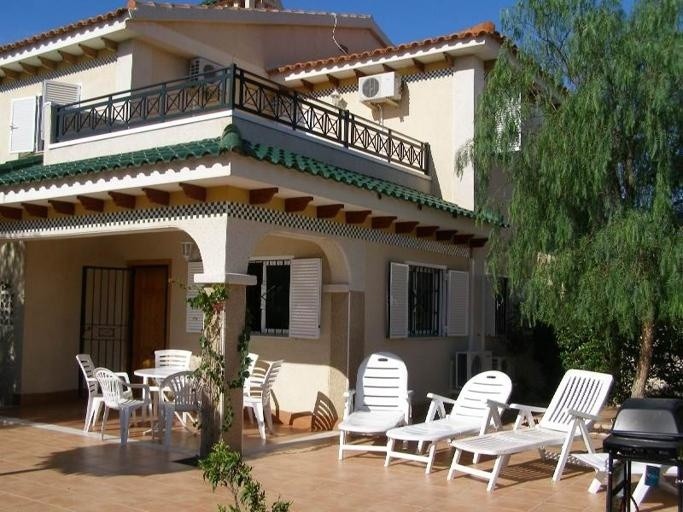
top-left (381, 366), bottom-right (511, 479)
top-left (343, 348), bottom-right (414, 466)
top-left (74, 349), bottom-right (284, 443)
top-left (455, 364), bottom-right (613, 489)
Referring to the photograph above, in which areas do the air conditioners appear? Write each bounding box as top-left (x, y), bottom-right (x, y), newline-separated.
top-left (357, 70), bottom-right (404, 107)
top-left (448, 349), bottom-right (493, 393)
top-left (491, 355), bottom-right (516, 388)
top-left (187, 57), bottom-right (225, 91)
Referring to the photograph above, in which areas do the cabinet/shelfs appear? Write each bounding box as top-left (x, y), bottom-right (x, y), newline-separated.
top-left (602, 434), bottom-right (682, 512)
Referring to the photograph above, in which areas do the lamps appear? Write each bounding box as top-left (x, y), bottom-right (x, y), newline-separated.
top-left (177, 233), bottom-right (193, 262)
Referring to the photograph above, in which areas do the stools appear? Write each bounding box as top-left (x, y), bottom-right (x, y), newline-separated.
top-left (571, 444), bottom-right (682, 512)
